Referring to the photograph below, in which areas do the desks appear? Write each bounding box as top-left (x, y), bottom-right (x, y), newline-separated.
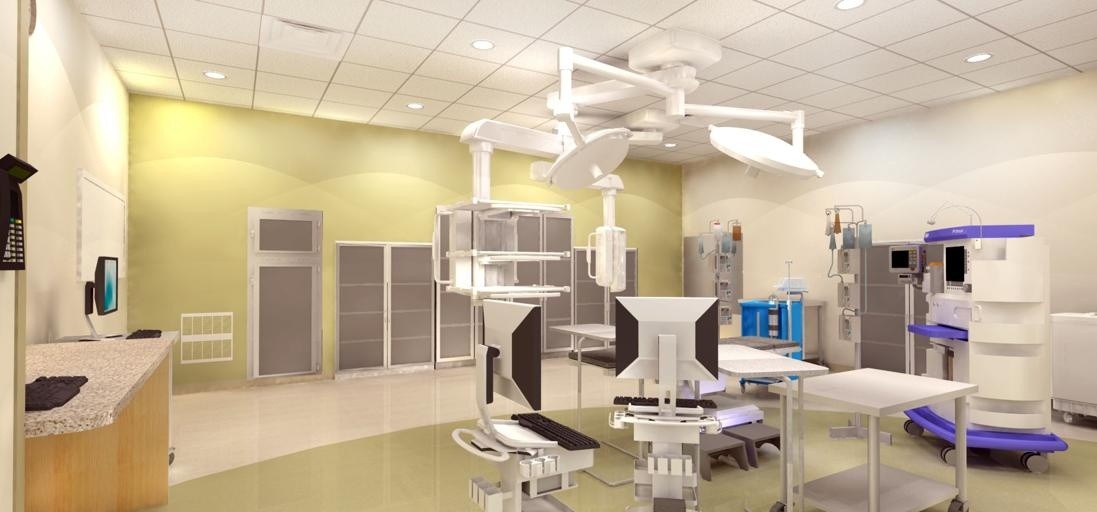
top-left (550, 323), bottom-right (616, 430)
top-left (718, 343), bottom-right (830, 512)
top-left (58, 330), bottom-right (181, 343)
top-left (25, 339), bottom-right (170, 512)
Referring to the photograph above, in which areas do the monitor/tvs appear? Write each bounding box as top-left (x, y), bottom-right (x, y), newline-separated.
top-left (475, 299), bottom-right (542, 431)
top-left (615, 296), bottom-right (719, 415)
top-left (78, 256), bottom-right (125, 341)
top-left (888, 246), bottom-right (922, 274)
top-left (946, 245), bottom-right (964, 282)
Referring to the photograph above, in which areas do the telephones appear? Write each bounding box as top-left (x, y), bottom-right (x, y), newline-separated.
top-left (0, 153), bottom-right (39, 270)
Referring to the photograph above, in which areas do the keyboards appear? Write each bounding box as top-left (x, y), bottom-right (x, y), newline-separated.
top-left (614, 396), bottom-right (717, 409)
top-left (126, 329), bottom-right (161, 339)
top-left (511, 413), bottom-right (600, 450)
top-left (25, 375), bottom-right (87, 411)
